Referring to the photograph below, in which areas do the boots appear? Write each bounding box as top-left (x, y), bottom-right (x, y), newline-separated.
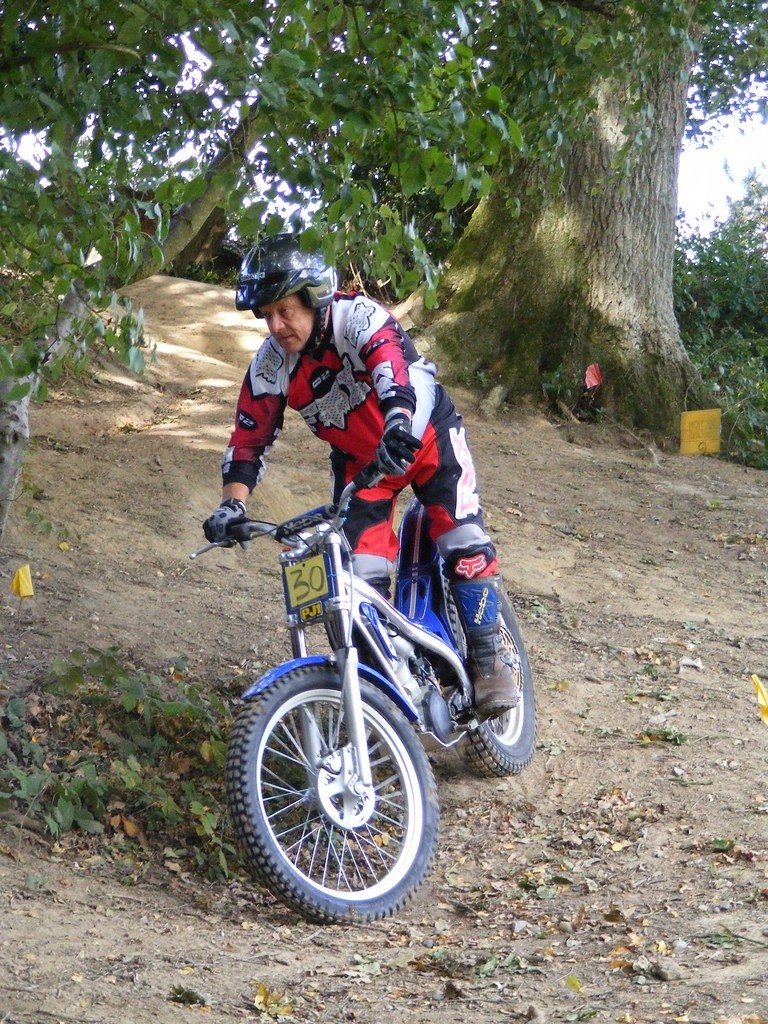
top-left (351, 577), bottom-right (392, 680)
top-left (450, 574), bottom-right (518, 713)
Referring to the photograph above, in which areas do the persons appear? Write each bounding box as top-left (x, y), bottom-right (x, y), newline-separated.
top-left (203, 235), bottom-right (520, 716)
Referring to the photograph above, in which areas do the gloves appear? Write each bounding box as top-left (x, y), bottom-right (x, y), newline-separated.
top-left (375, 407), bottom-right (424, 476)
top-left (203, 498), bottom-right (249, 550)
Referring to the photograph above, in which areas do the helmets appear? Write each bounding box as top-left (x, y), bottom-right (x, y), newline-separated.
top-left (236, 233), bottom-right (338, 318)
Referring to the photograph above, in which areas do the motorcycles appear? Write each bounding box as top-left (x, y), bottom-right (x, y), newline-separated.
top-left (187, 439), bottom-right (541, 926)
